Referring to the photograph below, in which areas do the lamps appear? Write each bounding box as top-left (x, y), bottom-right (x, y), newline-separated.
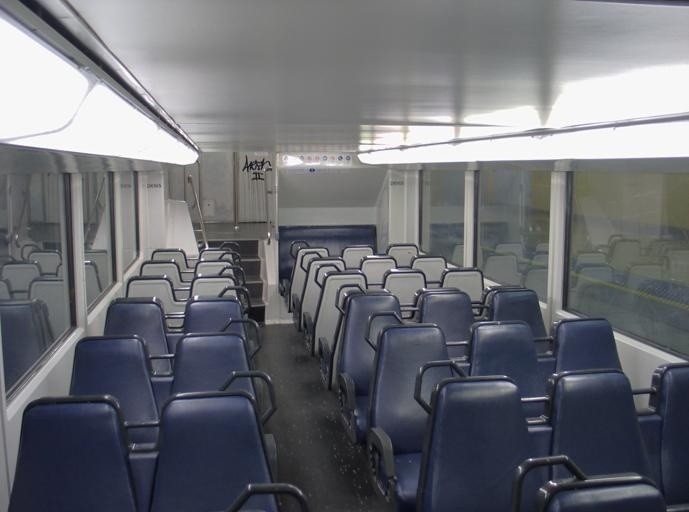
top-left (0, 1), bottom-right (201, 170)
top-left (355, 114), bottom-right (689, 173)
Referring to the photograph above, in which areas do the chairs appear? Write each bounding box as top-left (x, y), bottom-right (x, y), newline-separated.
top-left (0, 233), bottom-right (689, 512)
